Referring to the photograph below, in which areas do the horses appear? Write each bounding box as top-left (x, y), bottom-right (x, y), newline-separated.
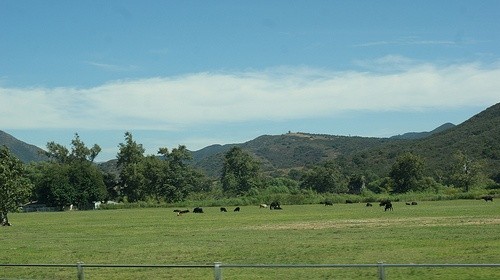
top-left (482, 196), bottom-right (494, 202)
top-left (172, 199), bottom-right (418, 215)
